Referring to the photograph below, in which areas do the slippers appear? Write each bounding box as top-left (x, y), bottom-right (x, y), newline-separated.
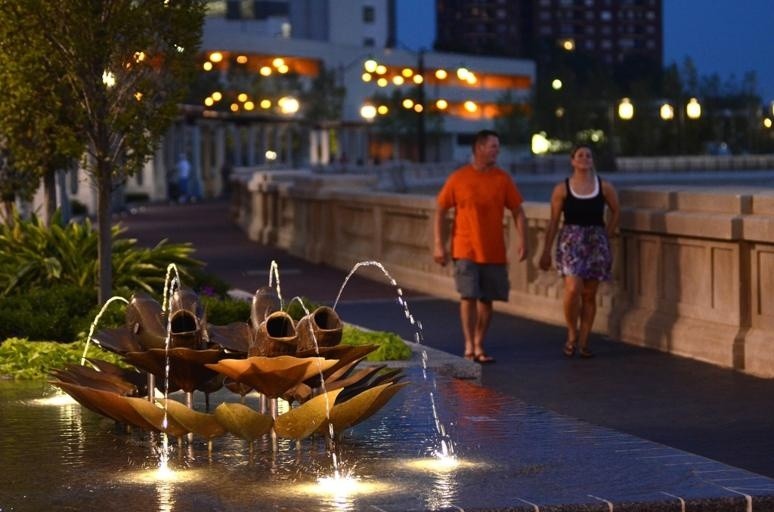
top-left (464, 353), bottom-right (496, 363)
top-left (562, 331), bottom-right (593, 358)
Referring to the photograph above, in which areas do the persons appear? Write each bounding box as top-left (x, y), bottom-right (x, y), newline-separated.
top-left (430, 128), bottom-right (528, 362)
top-left (539, 143), bottom-right (621, 359)
top-left (328, 150), bottom-right (382, 167)
top-left (174, 152), bottom-right (196, 202)
